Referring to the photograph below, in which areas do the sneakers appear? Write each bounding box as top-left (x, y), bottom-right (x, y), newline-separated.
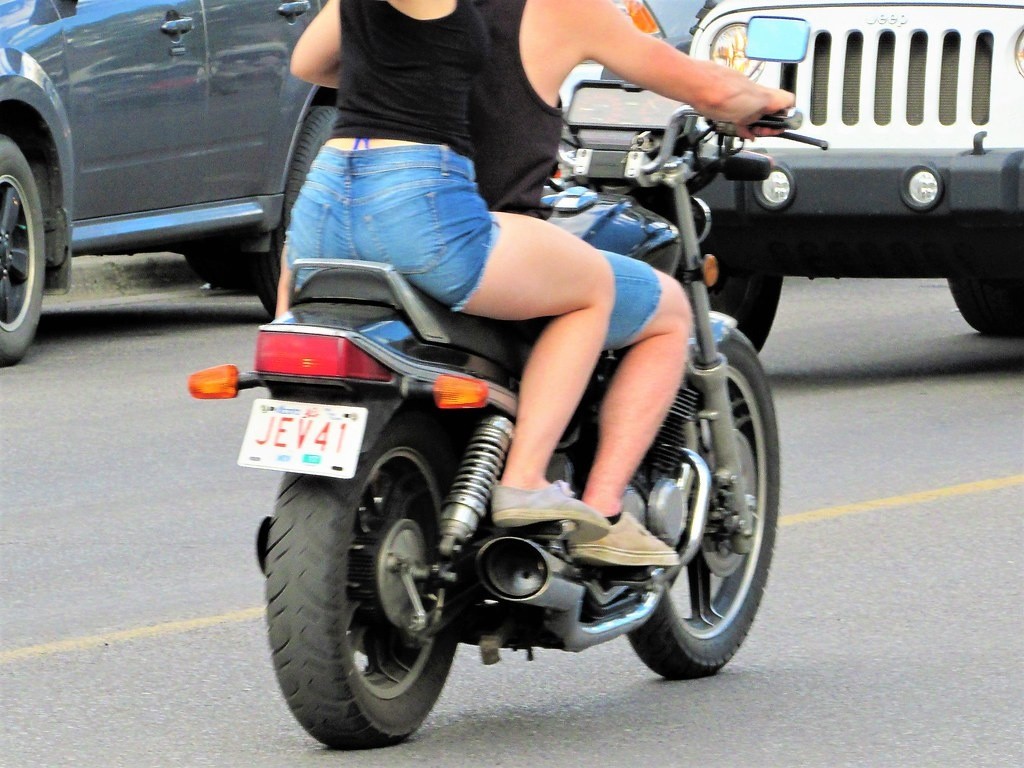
top-left (490, 477), bottom-right (610, 546)
top-left (574, 510), bottom-right (680, 571)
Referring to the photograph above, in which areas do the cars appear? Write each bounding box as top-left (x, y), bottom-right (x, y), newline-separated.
top-left (0, 0), bottom-right (341, 369)
top-left (552, 74), bottom-right (689, 198)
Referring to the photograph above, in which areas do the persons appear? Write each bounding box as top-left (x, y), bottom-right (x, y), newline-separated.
top-left (274, 0), bottom-right (616, 543)
top-left (465, 0), bottom-right (796, 567)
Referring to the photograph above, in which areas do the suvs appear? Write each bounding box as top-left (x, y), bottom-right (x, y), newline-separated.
top-left (654, 2), bottom-right (1024, 377)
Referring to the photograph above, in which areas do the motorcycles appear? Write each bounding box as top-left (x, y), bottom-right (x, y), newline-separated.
top-left (187, 12), bottom-right (831, 753)
